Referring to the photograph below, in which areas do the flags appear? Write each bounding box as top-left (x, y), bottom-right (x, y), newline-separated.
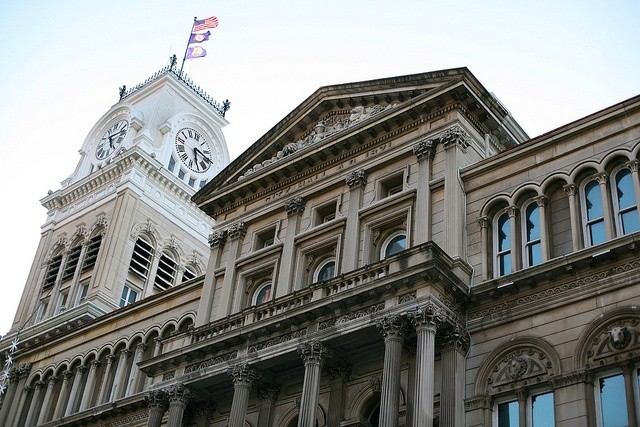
top-left (186, 46), bottom-right (207, 59)
top-left (192, 16), bottom-right (219, 31)
top-left (189, 31), bottom-right (211, 43)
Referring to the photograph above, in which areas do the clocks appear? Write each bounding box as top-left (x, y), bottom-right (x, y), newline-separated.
top-left (92, 116), bottom-right (130, 162)
top-left (171, 123), bottom-right (218, 179)
